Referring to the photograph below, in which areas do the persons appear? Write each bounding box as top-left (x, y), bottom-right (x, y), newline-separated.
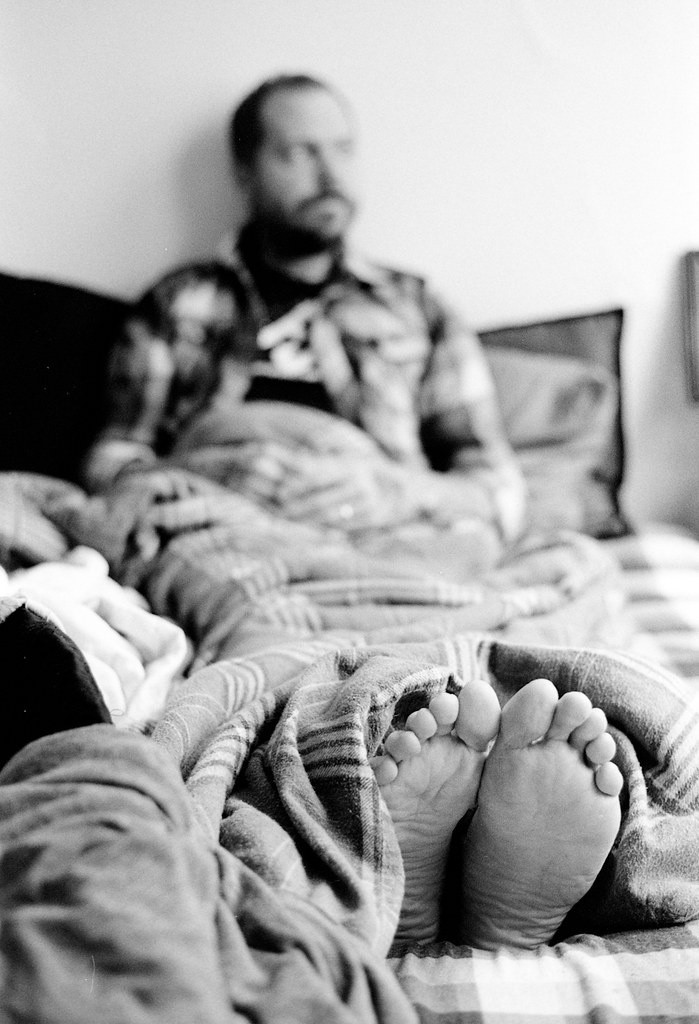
top-left (80, 72), bottom-right (625, 959)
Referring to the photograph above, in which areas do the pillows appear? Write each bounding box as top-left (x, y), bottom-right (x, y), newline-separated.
top-left (0, 270), bottom-right (633, 539)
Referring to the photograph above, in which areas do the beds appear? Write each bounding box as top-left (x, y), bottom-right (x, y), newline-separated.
top-left (0, 515), bottom-right (699, 1024)
